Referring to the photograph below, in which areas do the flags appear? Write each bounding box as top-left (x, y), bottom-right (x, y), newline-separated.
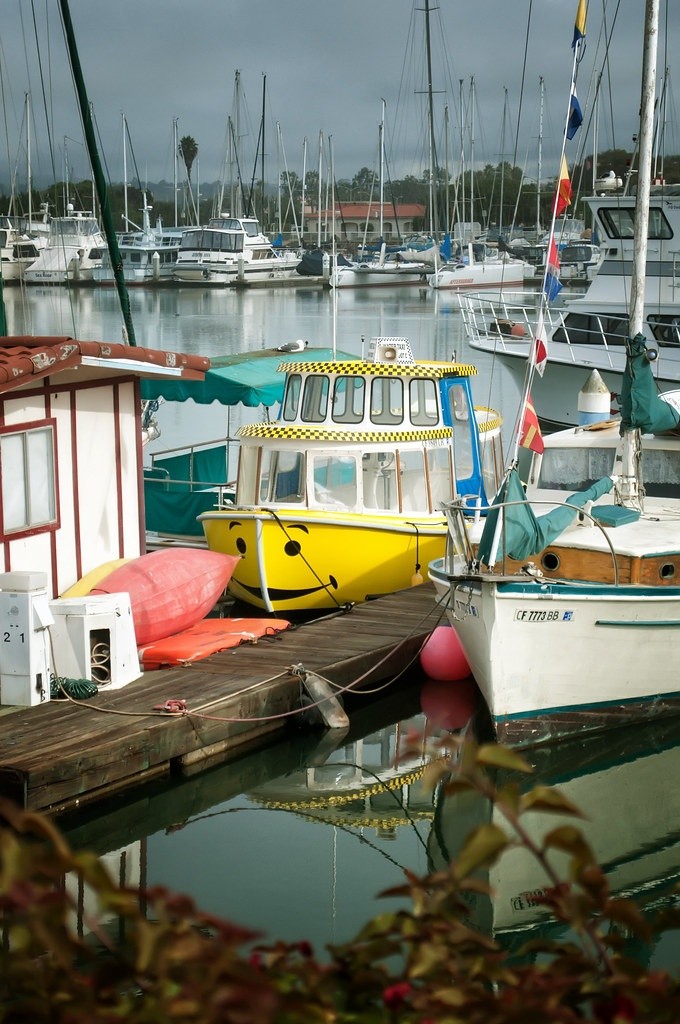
top-left (511, 0), bottom-right (590, 457)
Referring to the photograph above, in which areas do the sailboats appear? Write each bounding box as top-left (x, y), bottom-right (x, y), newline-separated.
top-left (1, 0), bottom-right (679, 747)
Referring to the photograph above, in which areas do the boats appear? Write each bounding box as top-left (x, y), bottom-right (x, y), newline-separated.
top-left (243, 708), bottom-right (485, 839)
top-left (140, 344), bottom-right (368, 550)
top-left (194, 334), bottom-right (505, 613)
top-left (426, 724), bottom-right (679, 939)
